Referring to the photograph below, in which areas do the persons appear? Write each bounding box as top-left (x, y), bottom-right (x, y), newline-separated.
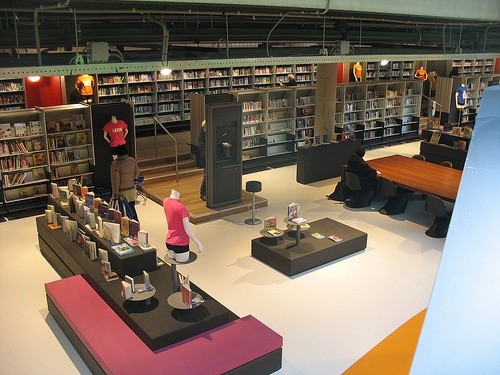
top-left (199, 120), bottom-right (206, 202)
top-left (353, 62), bottom-right (362, 83)
top-left (280, 74), bottom-right (297, 86)
top-left (415, 67), bottom-right (427, 81)
top-left (346, 148), bottom-right (378, 191)
top-left (111, 145), bottom-right (140, 223)
top-left (70, 82), bottom-right (86, 104)
top-left (103, 116), bottom-right (128, 160)
top-left (75, 74), bottom-right (94, 103)
top-left (455, 83), bottom-right (466, 108)
top-left (162, 189), bottom-right (203, 262)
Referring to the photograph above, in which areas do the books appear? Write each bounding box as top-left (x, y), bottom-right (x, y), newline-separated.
top-left (0, 115), bottom-right (93, 201)
top-left (462, 82), bottom-right (485, 115)
top-left (0, 82), bottom-right (22, 110)
top-left (426, 116), bottom-right (472, 150)
top-left (315, 127), bottom-right (345, 145)
top-left (345, 88), bottom-right (401, 140)
top-left (45, 178), bottom-right (193, 307)
top-left (263, 201), bottom-right (342, 244)
top-left (209, 64), bottom-right (318, 94)
top-left (97, 66), bottom-right (206, 126)
top-left (241, 96), bottom-right (314, 160)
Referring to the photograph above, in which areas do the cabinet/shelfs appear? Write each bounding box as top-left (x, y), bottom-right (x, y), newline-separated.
top-left (451, 73), bottom-right (500, 124)
top-left (36, 193), bottom-right (232, 351)
top-left (335, 77), bottom-right (424, 140)
top-left (420, 128), bottom-right (469, 169)
top-left (361, 61), bottom-right (416, 81)
top-left (0, 77), bottom-right (97, 207)
top-left (95, 64), bottom-right (318, 159)
top-left (446, 59), bottom-right (496, 76)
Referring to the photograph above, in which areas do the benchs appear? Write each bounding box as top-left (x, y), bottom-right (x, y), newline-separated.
top-left (44, 274), bottom-right (283, 375)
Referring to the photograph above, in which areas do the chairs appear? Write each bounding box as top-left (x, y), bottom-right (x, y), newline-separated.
top-left (412, 155), bottom-right (426, 160)
top-left (439, 161), bottom-right (452, 168)
top-left (344, 169), bottom-right (377, 207)
top-left (425, 193), bottom-right (452, 238)
top-left (380, 177), bottom-right (410, 215)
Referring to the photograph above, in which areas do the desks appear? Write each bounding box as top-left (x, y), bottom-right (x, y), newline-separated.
top-left (164, 251), bottom-right (204, 313)
top-left (296, 139), bottom-right (356, 184)
top-left (365, 154), bottom-right (463, 203)
top-left (252, 217), bottom-right (368, 276)
top-left (121, 283), bottom-right (157, 305)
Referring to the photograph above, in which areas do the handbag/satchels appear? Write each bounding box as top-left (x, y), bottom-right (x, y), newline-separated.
top-left (109, 195), bottom-right (135, 219)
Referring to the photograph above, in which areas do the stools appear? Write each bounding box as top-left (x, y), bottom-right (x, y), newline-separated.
top-left (244, 181), bottom-right (262, 226)
